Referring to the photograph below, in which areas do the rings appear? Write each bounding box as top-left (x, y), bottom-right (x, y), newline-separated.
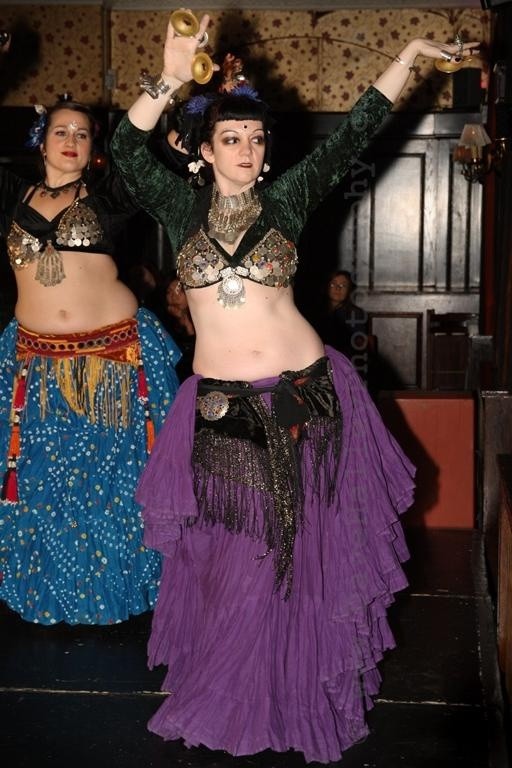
top-left (440, 50), bottom-right (452, 63)
top-left (453, 34), bottom-right (463, 54)
top-left (468, 48), bottom-right (472, 56)
top-left (192, 33), bottom-right (202, 42)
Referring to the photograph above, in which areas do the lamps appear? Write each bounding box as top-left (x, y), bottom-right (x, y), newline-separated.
top-left (451, 123), bottom-right (512, 184)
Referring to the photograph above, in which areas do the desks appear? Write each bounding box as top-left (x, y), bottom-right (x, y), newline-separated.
top-left (376, 392), bottom-right (475, 530)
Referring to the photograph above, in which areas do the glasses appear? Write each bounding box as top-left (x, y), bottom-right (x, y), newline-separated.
top-left (329, 280), bottom-right (348, 289)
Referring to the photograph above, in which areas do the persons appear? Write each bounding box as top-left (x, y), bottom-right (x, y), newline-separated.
top-left (151, 270), bottom-right (196, 379)
top-left (0, 22), bottom-right (192, 627)
top-left (325, 270), bottom-right (367, 372)
top-left (109, 3), bottom-right (487, 763)
top-left (127, 249), bottom-right (164, 314)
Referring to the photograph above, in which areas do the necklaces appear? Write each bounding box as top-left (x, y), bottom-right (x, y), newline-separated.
top-left (206, 181), bottom-right (260, 245)
top-left (35, 179), bottom-right (85, 199)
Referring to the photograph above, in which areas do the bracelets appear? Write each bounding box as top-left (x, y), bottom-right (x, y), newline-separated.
top-left (139, 68), bottom-right (179, 104)
top-left (394, 53), bottom-right (416, 72)
